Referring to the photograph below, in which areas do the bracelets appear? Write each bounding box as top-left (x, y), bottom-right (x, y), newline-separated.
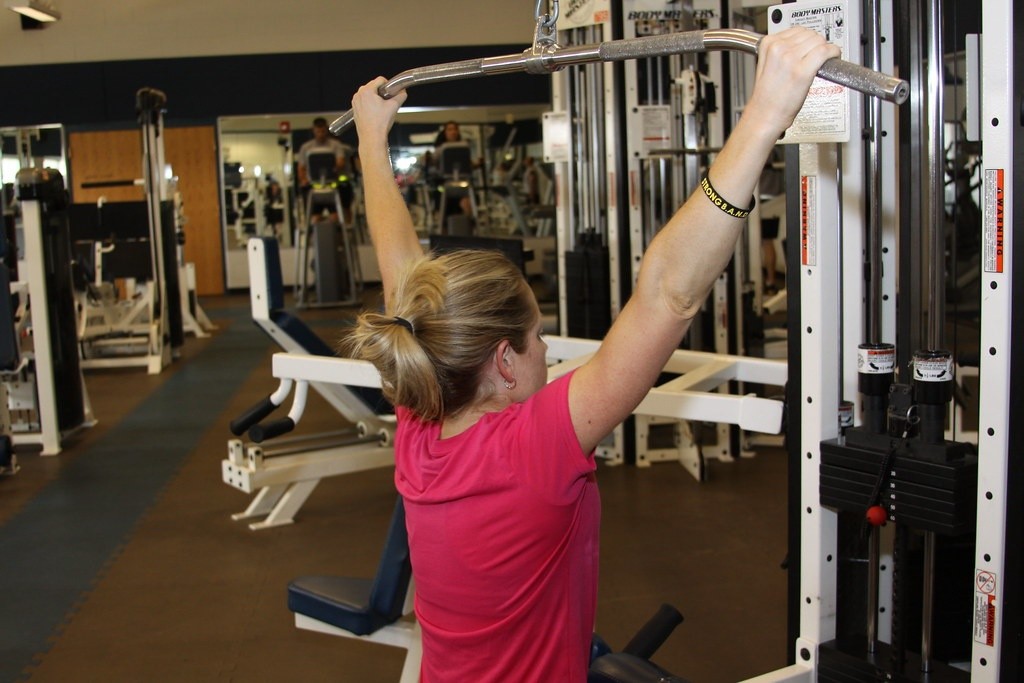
top-left (701, 168), bottom-right (756, 218)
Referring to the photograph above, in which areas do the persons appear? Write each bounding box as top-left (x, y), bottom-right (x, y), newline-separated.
top-left (352, 25), bottom-right (841, 683)
top-left (265, 175), bottom-right (281, 202)
top-left (298, 118), bottom-right (346, 223)
top-left (523, 155), bottom-right (541, 203)
top-left (760, 198), bottom-right (782, 295)
top-left (444, 119), bottom-right (480, 215)
top-left (492, 163), bottom-right (504, 185)
